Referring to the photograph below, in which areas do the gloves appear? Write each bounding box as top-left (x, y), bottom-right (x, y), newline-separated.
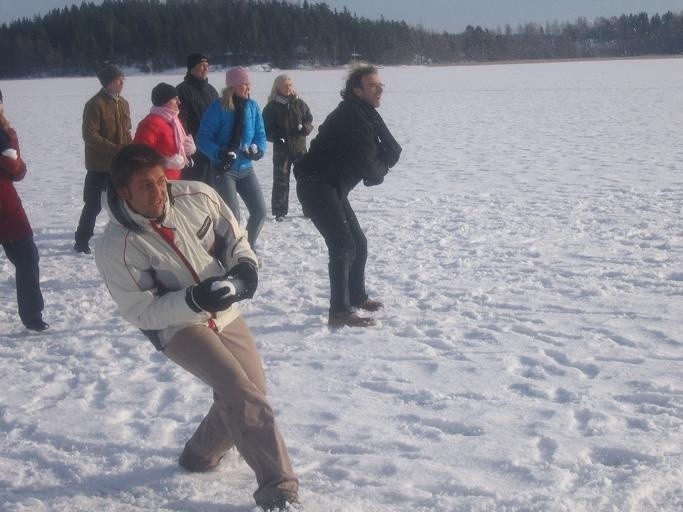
top-left (164, 158), bottom-right (186, 171)
top-left (240, 146), bottom-right (264, 160)
top-left (183, 136), bottom-right (197, 156)
top-left (183, 275), bottom-right (237, 313)
top-left (226, 261), bottom-right (258, 303)
top-left (1, 156), bottom-right (23, 178)
top-left (218, 150), bottom-right (235, 163)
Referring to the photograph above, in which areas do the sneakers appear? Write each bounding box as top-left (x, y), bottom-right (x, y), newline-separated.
top-left (74, 238), bottom-right (91, 253)
top-left (328, 312), bottom-right (376, 327)
top-left (263, 496), bottom-right (303, 511)
top-left (25, 321), bottom-right (49, 332)
top-left (356, 299), bottom-right (382, 312)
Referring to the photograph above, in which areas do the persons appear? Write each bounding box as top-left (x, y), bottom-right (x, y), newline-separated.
top-left (193, 65), bottom-right (268, 255)
top-left (294, 64), bottom-right (402, 330)
top-left (263, 74), bottom-right (313, 223)
top-left (0, 87), bottom-right (51, 332)
top-left (69, 62), bottom-right (134, 256)
top-left (173, 52), bottom-right (220, 187)
top-left (133, 80), bottom-right (198, 182)
top-left (95, 140), bottom-right (310, 512)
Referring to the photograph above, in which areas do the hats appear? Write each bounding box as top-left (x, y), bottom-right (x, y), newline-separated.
top-left (96, 60), bottom-right (125, 86)
top-left (150, 82), bottom-right (179, 107)
top-left (225, 68), bottom-right (250, 89)
top-left (187, 53), bottom-right (207, 70)
